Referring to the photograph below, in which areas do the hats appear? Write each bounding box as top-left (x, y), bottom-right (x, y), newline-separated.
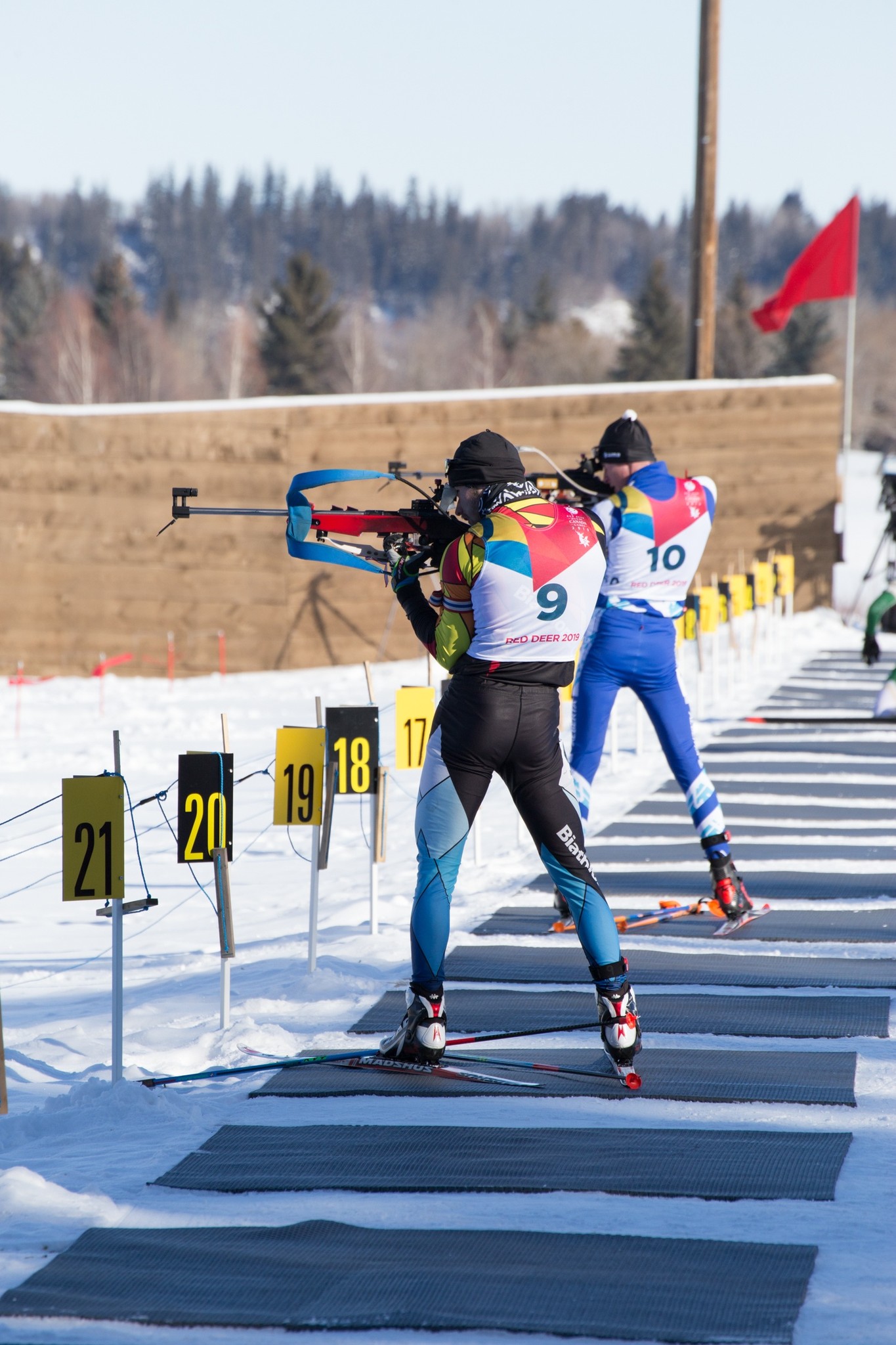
top-left (596, 416), bottom-right (661, 464)
top-left (444, 430), bottom-right (530, 486)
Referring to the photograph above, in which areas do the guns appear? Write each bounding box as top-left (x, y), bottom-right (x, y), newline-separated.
top-left (376, 446), bottom-right (616, 518)
top-left (156, 469), bottom-right (473, 583)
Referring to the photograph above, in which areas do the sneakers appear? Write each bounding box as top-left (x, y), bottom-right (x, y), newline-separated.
top-left (380, 986), bottom-right (448, 1065)
top-left (596, 986), bottom-right (644, 1061)
top-left (553, 884), bottom-right (569, 918)
top-left (711, 856), bottom-right (753, 917)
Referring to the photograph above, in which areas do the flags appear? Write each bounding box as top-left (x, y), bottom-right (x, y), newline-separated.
top-left (751, 195), bottom-right (870, 334)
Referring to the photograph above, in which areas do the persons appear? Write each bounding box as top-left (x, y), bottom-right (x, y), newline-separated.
top-left (379, 427), bottom-right (644, 1066)
top-left (546, 412), bottom-right (757, 924)
